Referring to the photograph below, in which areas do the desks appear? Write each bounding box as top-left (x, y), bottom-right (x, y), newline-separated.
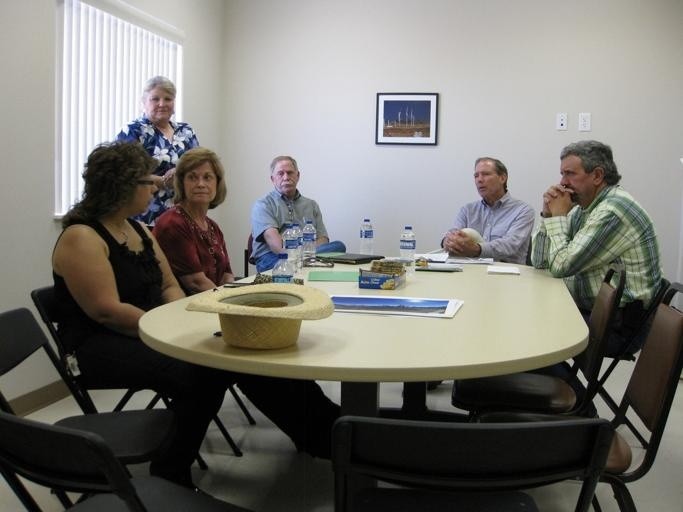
top-left (138, 253), bottom-right (590, 506)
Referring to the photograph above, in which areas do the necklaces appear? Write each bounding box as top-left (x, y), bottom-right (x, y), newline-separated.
top-left (100, 219), bottom-right (130, 239)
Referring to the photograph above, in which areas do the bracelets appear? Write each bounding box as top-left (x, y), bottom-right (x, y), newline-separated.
top-left (160, 175), bottom-right (169, 193)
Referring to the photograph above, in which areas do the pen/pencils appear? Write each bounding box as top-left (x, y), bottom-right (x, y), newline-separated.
top-left (224, 286), bottom-right (236, 288)
top-left (213, 332), bottom-right (223, 337)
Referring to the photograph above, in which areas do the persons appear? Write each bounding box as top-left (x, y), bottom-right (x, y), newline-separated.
top-left (116, 77), bottom-right (200, 223)
top-left (425, 158), bottom-right (536, 393)
top-left (50, 142), bottom-right (229, 488)
top-left (248, 155), bottom-right (347, 273)
top-left (153, 148), bottom-right (344, 463)
top-left (531, 139), bottom-right (665, 378)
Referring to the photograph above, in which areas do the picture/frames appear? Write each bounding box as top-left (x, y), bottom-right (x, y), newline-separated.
top-left (373, 92), bottom-right (439, 146)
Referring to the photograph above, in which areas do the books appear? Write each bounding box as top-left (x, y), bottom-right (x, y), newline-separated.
top-left (327, 250), bottom-right (385, 266)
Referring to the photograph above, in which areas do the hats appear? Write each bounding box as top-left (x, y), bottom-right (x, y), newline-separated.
top-left (185, 280), bottom-right (334, 350)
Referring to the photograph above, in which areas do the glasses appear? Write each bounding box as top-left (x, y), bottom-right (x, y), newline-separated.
top-left (136, 178), bottom-right (153, 189)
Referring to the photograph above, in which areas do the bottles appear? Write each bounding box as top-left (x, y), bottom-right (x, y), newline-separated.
top-left (291, 221), bottom-right (305, 271)
top-left (358, 218), bottom-right (375, 256)
top-left (398, 225), bottom-right (416, 280)
top-left (271, 252), bottom-right (295, 284)
top-left (280, 224), bottom-right (298, 273)
top-left (301, 220), bottom-right (317, 265)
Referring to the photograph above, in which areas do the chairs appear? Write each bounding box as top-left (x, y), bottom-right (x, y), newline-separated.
top-left (573, 278), bottom-right (670, 451)
top-left (0, 413), bottom-right (250, 512)
top-left (0, 307), bottom-right (176, 511)
top-left (243, 234), bottom-right (260, 277)
top-left (450, 267), bottom-right (629, 419)
top-left (30, 285), bottom-right (259, 471)
top-left (331, 414), bottom-right (611, 510)
top-left (470, 282), bottom-right (681, 510)
